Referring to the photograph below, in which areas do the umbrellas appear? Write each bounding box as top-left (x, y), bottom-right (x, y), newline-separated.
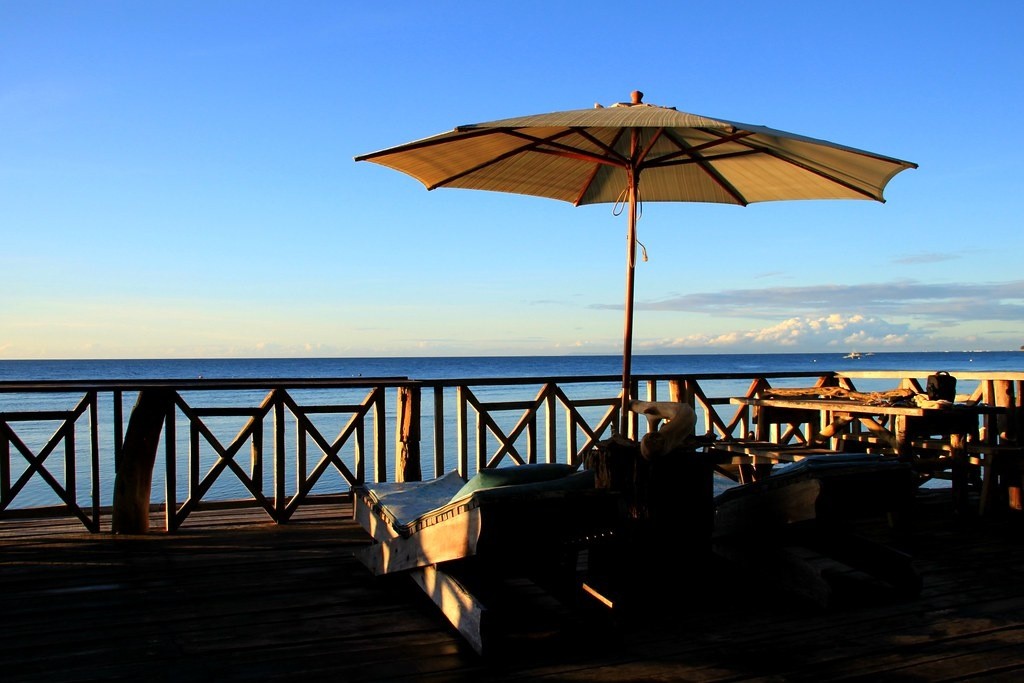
top-left (354, 91), bottom-right (920, 443)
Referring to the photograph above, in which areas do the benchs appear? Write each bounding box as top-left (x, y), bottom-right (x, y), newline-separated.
top-left (710, 437), bottom-right (1023, 523)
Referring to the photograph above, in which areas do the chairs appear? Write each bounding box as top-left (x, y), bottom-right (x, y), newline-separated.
top-left (348, 463), bottom-right (619, 659)
top-left (702, 448), bottom-right (922, 617)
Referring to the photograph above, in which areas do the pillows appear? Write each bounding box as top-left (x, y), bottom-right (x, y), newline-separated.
top-left (447, 464), bottom-right (579, 504)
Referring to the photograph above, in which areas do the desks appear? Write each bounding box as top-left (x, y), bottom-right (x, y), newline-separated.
top-left (729, 396), bottom-right (1014, 516)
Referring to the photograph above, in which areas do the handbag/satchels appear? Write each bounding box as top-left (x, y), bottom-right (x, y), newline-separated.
top-left (927, 371), bottom-right (956, 403)
